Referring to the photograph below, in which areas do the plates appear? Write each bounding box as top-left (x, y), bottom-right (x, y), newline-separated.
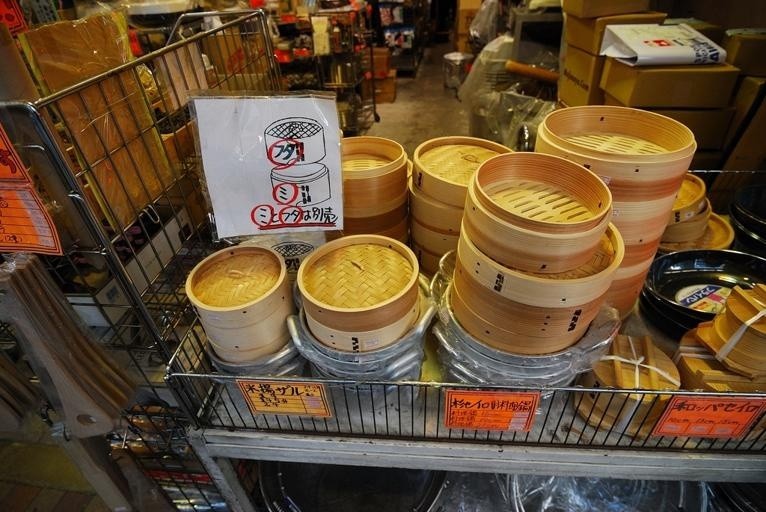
top-left (636, 246), bottom-right (765, 341)
top-left (724, 181), bottom-right (766, 249)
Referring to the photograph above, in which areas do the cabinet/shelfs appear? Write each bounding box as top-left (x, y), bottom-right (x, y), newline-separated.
top-left (276, 1), bottom-right (380, 143)
top-left (0, 7), bottom-right (274, 511)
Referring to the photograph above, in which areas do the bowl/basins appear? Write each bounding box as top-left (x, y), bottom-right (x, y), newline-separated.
top-left (285, 272), bottom-right (436, 392)
top-left (430, 247), bottom-right (622, 414)
top-left (257, 460), bottom-right (450, 511)
